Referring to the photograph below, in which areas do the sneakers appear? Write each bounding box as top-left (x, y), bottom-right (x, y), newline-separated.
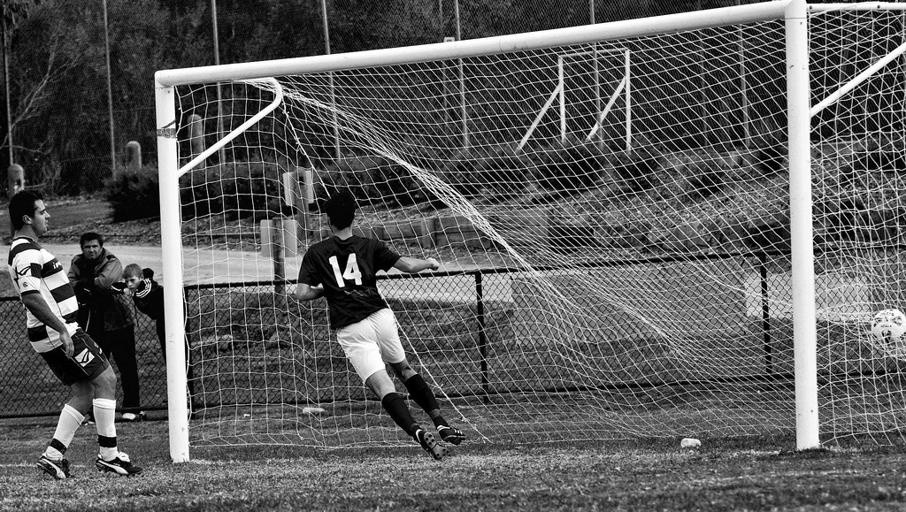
top-left (94, 452), bottom-right (144, 477)
top-left (122, 411), bottom-right (140, 421)
top-left (415, 428), bottom-right (451, 460)
top-left (36, 452), bottom-right (75, 479)
top-left (437, 424), bottom-right (467, 446)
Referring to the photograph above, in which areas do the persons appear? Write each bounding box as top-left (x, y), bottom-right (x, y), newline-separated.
top-left (297, 191), bottom-right (466, 460)
top-left (110, 263), bottom-right (195, 421)
top-left (7, 189), bottom-right (143, 480)
top-left (66, 231), bottom-right (147, 424)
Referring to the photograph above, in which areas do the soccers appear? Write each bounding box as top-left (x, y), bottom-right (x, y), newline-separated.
top-left (871, 309), bottom-right (906, 342)
